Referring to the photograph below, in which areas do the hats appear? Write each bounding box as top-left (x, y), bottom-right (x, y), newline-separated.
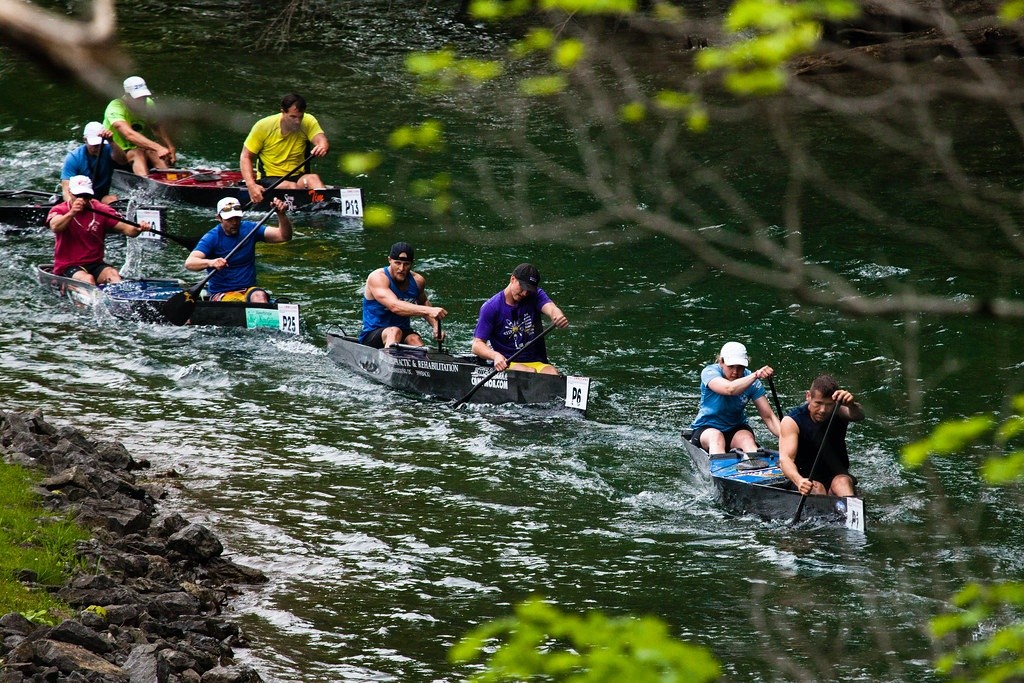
top-left (512, 264), bottom-right (539, 293)
top-left (217, 197), bottom-right (244, 219)
top-left (389, 242), bottom-right (414, 262)
top-left (123, 76), bottom-right (152, 99)
top-left (720, 342), bottom-right (749, 368)
top-left (83, 121), bottom-right (109, 145)
top-left (68, 176), bottom-right (94, 196)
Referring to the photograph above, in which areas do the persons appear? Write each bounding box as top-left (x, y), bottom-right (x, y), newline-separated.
top-left (779, 375), bottom-right (865, 497)
top-left (61, 122), bottom-right (131, 207)
top-left (185, 197), bottom-right (292, 304)
top-left (472, 263), bottom-right (570, 374)
top-left (241, 95), bottom-right (329, 202)
top-left (689, 342), bottom-right (781, 459)
top-left (46, 174), bottom-right (150, 286)
top-left (359, 243), bottom-right (447, 349)
top-left (103, 76), bottom-right (175, 180)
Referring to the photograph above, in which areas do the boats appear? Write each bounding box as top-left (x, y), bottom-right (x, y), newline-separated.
top-left (681, 431), bottom-right (868, 535)
top-left (113, 156), bottom-right (364, 221)
top-left (326, 328), bottom-right (591, 411)
top-left (36, 263), bottom-right (302, 336)
top-left (0, 189), bottom-right (169, 238)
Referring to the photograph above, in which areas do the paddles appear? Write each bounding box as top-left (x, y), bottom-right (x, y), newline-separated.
top-left (92, 137), bottom-right (106, 189)
top-left (770, 378), bottom-right (784, 422)
top-left (241, 154), bottom-right (316, 211)
top-left (163, 206), bottom-right (277, 327)
top-left (450, 323), bottom-right (557, 408)
top-left (438, 319), bottom-right (441, 352)
top-left (83, 205), bottom-right (203, 252)
top-left (787, 399), bottom-right (841, 527)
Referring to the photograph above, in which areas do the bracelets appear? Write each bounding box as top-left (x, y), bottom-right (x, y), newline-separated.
top-left (755, 370), bottom-right (761, 379)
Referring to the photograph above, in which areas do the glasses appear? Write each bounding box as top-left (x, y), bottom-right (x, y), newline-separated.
top-left (71, 192), bottom-right (93, 202)
top-left (217, 204), bottom-right (242, 217)
